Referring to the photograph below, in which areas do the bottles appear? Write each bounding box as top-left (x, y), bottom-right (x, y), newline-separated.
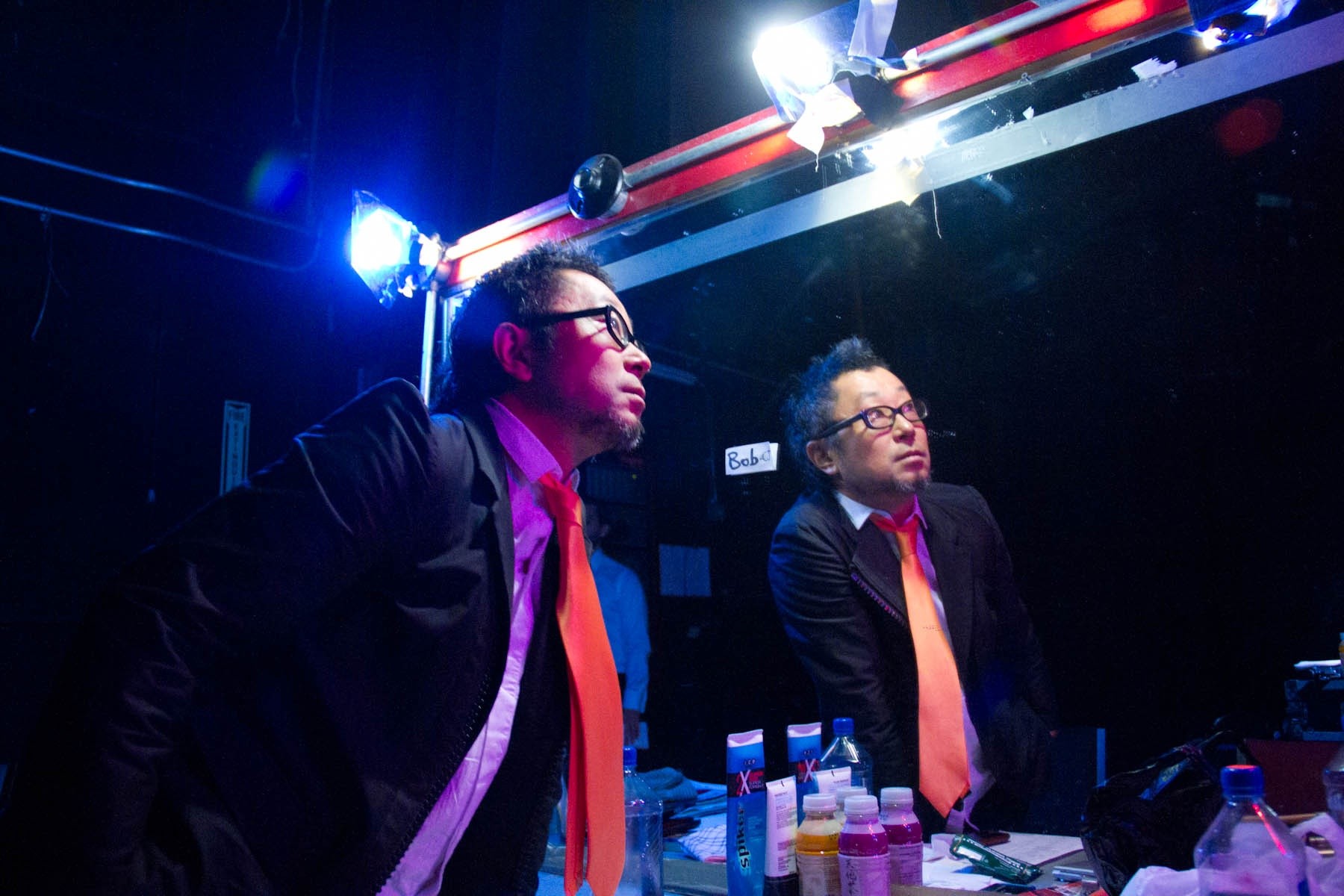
top-left (1321, 717), bottom-right (1344, 834)
top-left (837, 795), bottom-right (890, 896)
top-left (878, 787), bottom-right (923, 888)
top-left (795, 793), bottom-right (843, 896)
top-left (615, 746), bottom-right (663, 896)
top-left (833, 786), bottom-right (868, 828)
top-left (819, 716), bottom-right (873, 795)
top-left (1194, 764), bottom-right (1311, 896)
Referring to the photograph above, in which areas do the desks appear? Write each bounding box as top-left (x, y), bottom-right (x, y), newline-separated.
top-left (540, 779), bottom-right (1108, 896)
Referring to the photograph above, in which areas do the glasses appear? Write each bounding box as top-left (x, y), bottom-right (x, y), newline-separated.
top-left (512, 304), bottom-right (648, 356)
top-left (799, 399), bottom-right (928, 465)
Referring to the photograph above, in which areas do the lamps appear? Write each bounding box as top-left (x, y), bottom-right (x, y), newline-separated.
top-left (343, 189), bottom-right (451, 310)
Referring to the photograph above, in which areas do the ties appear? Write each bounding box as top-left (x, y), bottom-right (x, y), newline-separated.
top-left (539, 471), bottom-right (626, 896)
top-left (871, 513), bottom-right (970, 819)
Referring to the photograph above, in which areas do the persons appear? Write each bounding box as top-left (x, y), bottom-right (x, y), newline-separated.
top-left (86, 243), bottom-right (652, 896)
top-left (581, 492), bottom-right (648, 743)
top-left (769, 335), bottom-right (1059, 851)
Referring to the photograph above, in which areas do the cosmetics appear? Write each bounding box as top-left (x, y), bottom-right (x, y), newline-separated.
top-left (765, 775), bottom-right (797, 896)
top-left (722, 731), bottom-right (767, 896)
top-left (783, 722), bottom-right (820, 826)
top-left (817, 769), bottom-right (853, 791)
